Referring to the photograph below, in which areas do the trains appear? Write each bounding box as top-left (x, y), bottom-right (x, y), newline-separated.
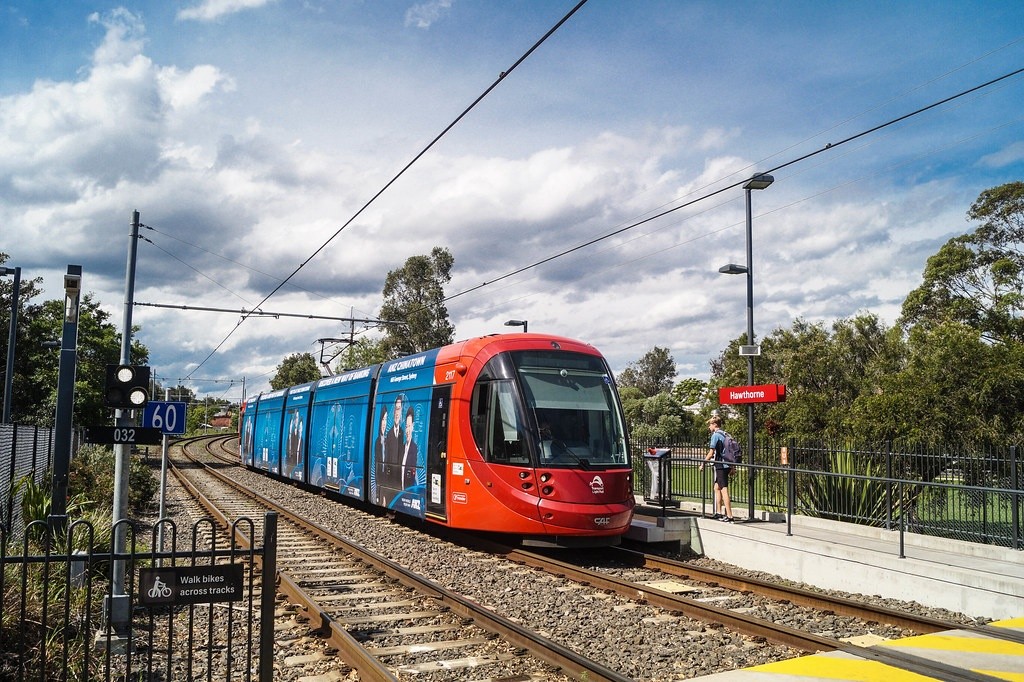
top-left (238, 331), bottom-right (635, 550)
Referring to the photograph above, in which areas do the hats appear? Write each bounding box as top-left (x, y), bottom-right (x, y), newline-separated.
top-left (706, 416), bottom-right (722, 423)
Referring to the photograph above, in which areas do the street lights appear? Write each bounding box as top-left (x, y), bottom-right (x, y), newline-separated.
top-left (504, 319), bottom-right (528, 332)
top-left (720, 173), bottom-right (774, 526)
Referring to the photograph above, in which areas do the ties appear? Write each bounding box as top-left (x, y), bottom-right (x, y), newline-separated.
top-left (396, 429), bottom-right (398, 438)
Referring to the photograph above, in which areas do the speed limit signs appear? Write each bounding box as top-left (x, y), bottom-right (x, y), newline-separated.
top-left (142, 400), bottom-right (186, 435)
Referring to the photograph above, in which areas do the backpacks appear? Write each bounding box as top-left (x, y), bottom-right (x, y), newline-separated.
top-left (715, 431), bottom-right (742, 466)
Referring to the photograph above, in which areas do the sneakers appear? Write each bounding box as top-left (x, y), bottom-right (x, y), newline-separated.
top-left (709, 513), bottom-right (722, 519)
top-left (719, 515), bottom-right (735, 524)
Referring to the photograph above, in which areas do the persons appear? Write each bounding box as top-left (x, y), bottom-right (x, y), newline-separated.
top-left (287, 411), bottom-right (302, 473)
top-left (386, 395), bottom-right (404, 488)
top-left (400, 407), bottom-right (418, 490)
top-left (375, 405), bottom-right (388, 486)
top-left (245, 417), bottom-right (252, 453)
top-left (700, 416), bottom-right (736, 524)
